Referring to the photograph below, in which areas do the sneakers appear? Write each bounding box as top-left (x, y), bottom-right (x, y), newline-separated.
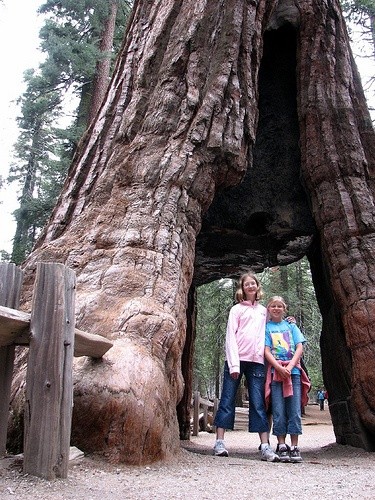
top-left (259, 444), bottom-right (280, 462)
top-left (212, 442), bottom-right (228, 457)
top-left (290, 448), bottom-right (302, 462)
top-left (277, 444), bottom-right (291, 463)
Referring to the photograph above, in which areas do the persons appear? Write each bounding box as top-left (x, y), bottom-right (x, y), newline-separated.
top-left (258, 296), bottom-right (311, 463)
top-left (315, 385), bottom-right (328, 411)
top-left (211, 272), bottom-right (281, 462)
